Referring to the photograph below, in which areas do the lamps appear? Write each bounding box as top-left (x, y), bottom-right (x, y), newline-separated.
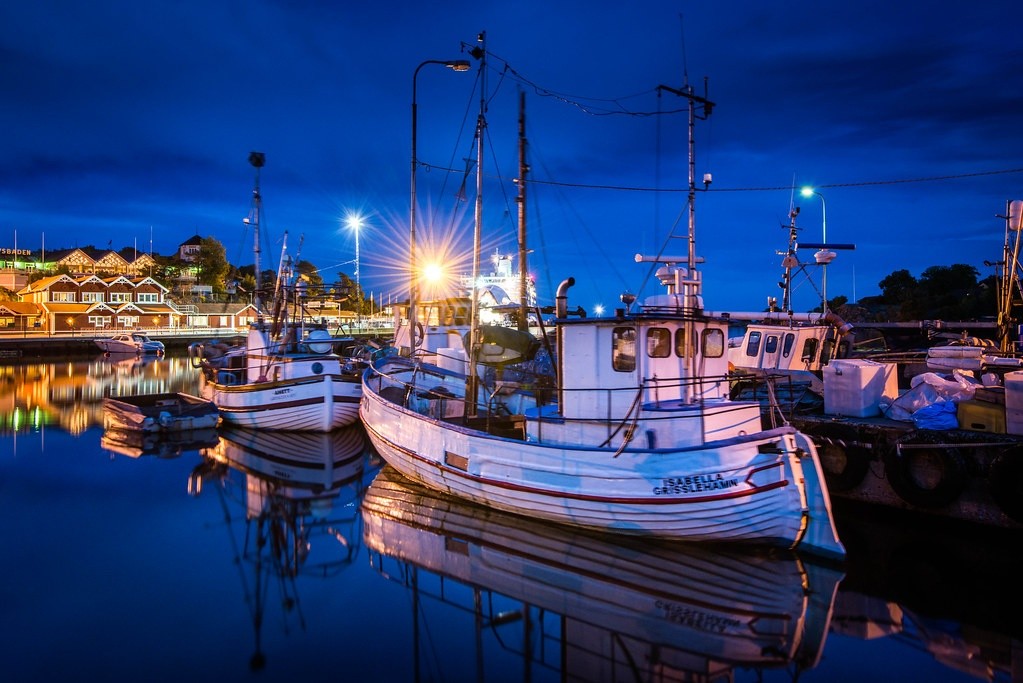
top-left (777, 281), bottom-right (785, 289)
top-left (620, 291), bottom-right (635, 315)
top-left (788, 310), bottom-right (793, 328)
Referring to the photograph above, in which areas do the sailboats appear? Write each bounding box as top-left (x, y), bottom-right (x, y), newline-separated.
top-left (358, 8), bottom-right (848, 559)
top-left (359, 462), bottom-right (847, 683)
top-left (187, 149), bottom-right (364, 434)
top-left (184, 416), bottom-right (382, 676)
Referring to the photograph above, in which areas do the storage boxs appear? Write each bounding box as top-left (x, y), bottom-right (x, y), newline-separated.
top-left (822, 358), bottom-right (899, 417)
top-left (957, 387), bottom-right (1006, 433)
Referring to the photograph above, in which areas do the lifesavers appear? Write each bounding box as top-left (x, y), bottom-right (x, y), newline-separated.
top-left (409, 320), bottom-right (425, 349)
top-left (986, 445), bottom-right (1023, 521)
top-left (882, 431), bottom-right (967, 515)
top-left (806, 423), bottom-right (869, 492)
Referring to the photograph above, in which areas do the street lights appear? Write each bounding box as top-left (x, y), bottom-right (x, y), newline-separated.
top-left (408, 56), bottom-right (475, 363)
top-left (798, 185), bottom-right (828, 312)
top-left (346, 216), bottom-right (360, 326)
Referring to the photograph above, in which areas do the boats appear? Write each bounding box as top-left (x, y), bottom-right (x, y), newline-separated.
top-left (99, 426), bottom-right (219, 461)
top-left (99, 392), bottom-right (224, 435)
top-left (728, 169), bottom-right (856, 378)
top-left (946, 199), bottom-right (1023, 361)
top-left (93, 331), bottom-right (165, 352)
